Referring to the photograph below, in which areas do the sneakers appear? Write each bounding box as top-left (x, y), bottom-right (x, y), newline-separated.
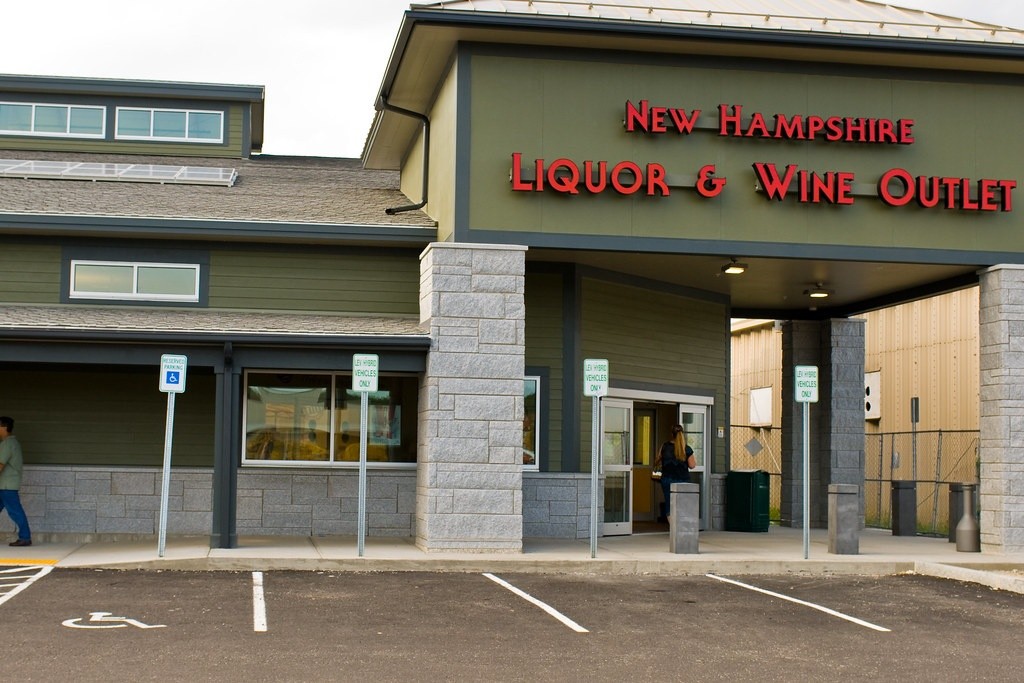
top-left (9, 539), bottom-right (32, 546)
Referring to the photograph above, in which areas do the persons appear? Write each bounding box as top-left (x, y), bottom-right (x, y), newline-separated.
top-left (0, 417), bottom-right (32, 546)
top-left (654, 424), bottom-right (695, 528)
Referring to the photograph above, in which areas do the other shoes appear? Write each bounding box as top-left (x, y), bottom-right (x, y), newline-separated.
top-left (666, 513), bottom-right (670, 524)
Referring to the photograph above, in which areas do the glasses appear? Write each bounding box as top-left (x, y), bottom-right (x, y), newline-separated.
top-left (342, 422), bottom-right (350, 443)
top-left (308, 420), bottom-right (317, 442)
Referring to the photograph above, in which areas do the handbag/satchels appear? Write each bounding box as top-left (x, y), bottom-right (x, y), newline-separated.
top-left (652, 461), bottom-right (663, 481)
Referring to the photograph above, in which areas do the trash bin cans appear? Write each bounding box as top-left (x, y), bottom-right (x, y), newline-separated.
top-left (725, 469), bottom-right (771, 532)
